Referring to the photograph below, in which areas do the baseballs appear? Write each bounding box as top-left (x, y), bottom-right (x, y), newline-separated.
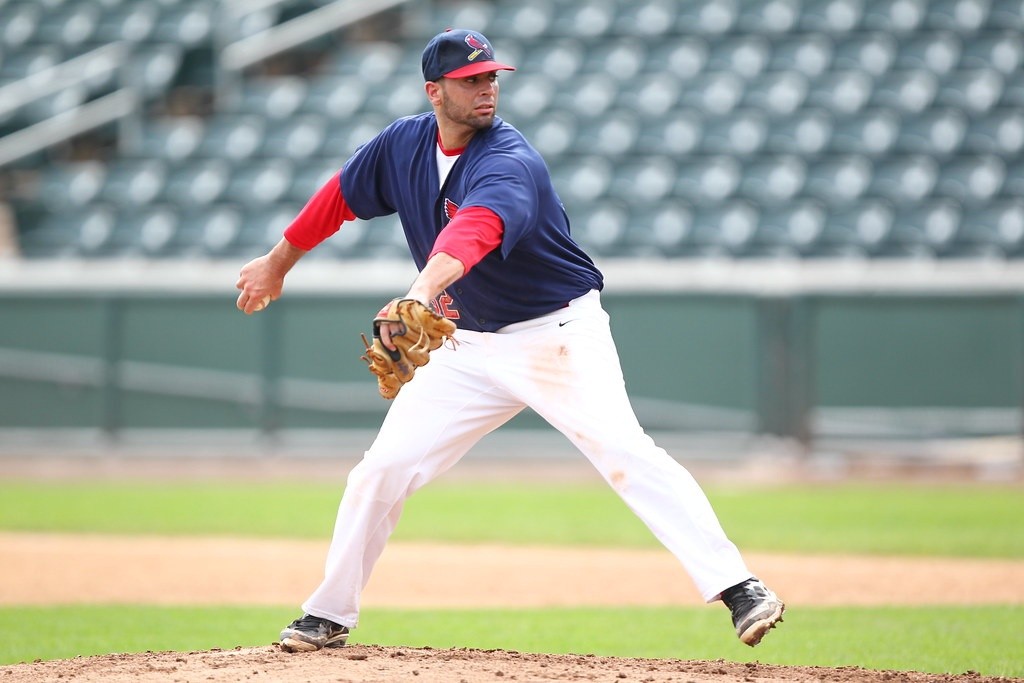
top-left (253, 294), bottom-right (270, 312)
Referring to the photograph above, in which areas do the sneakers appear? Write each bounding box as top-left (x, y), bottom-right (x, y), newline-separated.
top-left (721, 576), bottom-right (786, 647)
top-left (279, 613), bottom-right (349, 651)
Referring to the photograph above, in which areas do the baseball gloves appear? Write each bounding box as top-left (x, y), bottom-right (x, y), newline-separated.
top-left (360, 299), bottom-right (457, 400)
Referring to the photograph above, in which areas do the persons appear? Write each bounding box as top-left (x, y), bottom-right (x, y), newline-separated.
top-left (236, 27), bottom-right (784, 654)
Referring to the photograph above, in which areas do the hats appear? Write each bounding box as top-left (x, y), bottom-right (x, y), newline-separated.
top-left (421, 28), bottom-right (515, 82)
